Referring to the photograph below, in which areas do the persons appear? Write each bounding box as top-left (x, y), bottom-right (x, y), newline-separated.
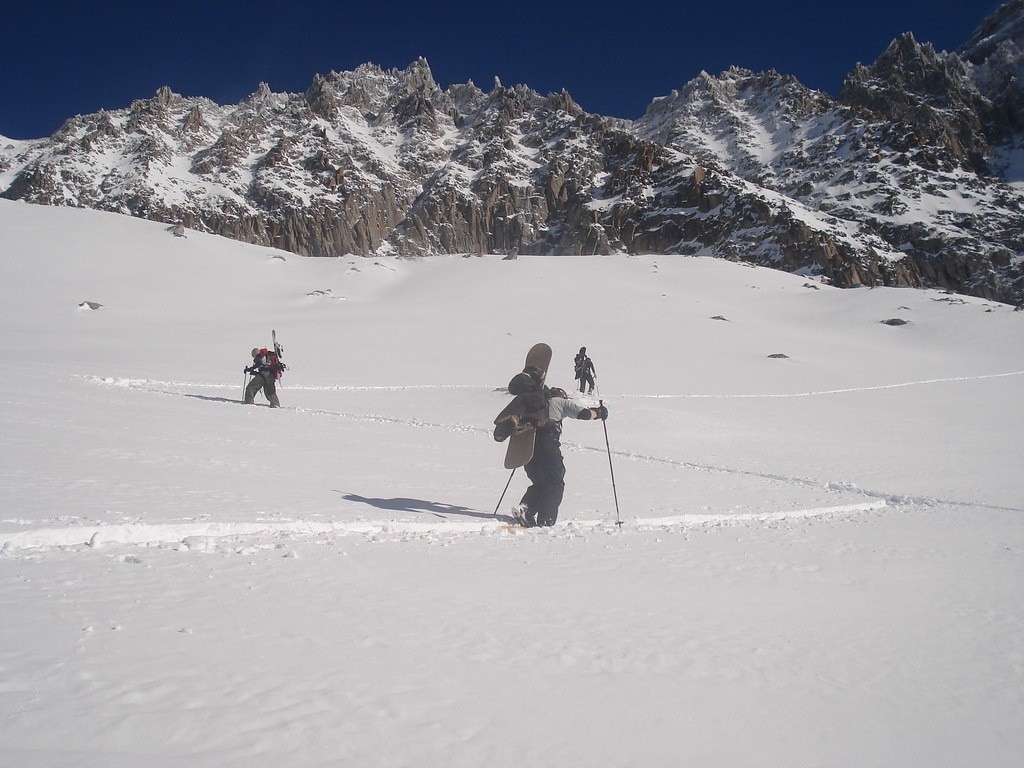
top-left (580, 355), bottom-right (597, 395)
top-left (510, 366), bottom-right (608, 527)
top-left (242, 348), bottom-right (280, 408)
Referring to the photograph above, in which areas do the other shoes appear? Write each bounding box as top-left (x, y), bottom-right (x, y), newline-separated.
top-left (512, 503), bottom-right (536, 527)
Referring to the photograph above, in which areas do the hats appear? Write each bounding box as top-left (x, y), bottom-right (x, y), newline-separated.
top-left (523, 366), bottom-right (545, 387)
top-left (251, 348), bottom-right (259, 357)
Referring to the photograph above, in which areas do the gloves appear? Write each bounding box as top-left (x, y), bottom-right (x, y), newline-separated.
top-left (244, 368), bottom-right (250, 373)
top-left (592, 406), bottom-right (608, 420)
top-left (251, 371), bottom-right (256, 375)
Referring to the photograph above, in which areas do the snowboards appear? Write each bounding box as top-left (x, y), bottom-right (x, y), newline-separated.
top-left (503, 342), bottom-right (554, 471)
top-left (573, 347), bottom-right (587, 380)
top-left (271, 329), bottom-right (283, 389)
